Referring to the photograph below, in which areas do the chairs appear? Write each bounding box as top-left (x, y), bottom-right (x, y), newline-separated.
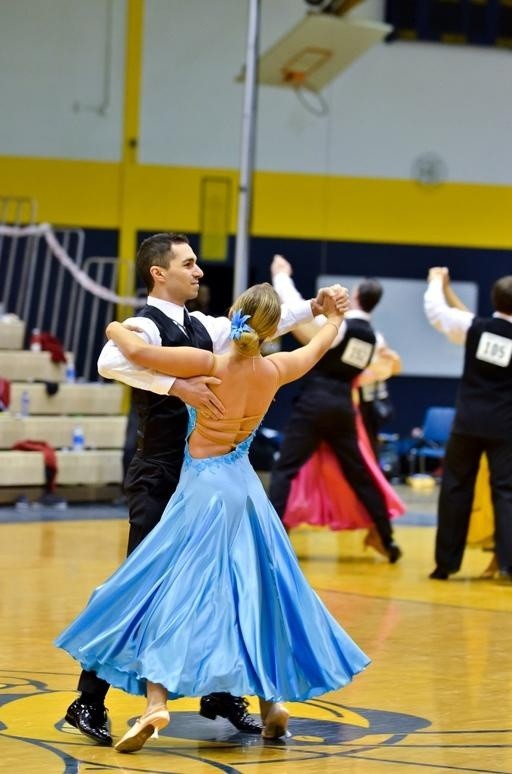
top-left (406, 405), bottom-right (457, 473)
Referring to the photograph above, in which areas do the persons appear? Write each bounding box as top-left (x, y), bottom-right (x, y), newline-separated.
top-left (424, 268), bottom-right (511, 580)
top-left (54, 283), bottom-right (371, 753)
top-left (64, 232), bottom-right (350, 745)
top-left (266, 254), bottom-right (407, 564)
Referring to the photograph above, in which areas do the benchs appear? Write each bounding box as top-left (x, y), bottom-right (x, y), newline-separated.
top-left (0, 305), bottom-right (130, 506)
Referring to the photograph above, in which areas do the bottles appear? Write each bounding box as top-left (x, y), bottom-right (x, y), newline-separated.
top-left (30, 325), bottom-right (42, 352)
top-left (73, 418), bottom-right (86, 449)
top-left (66, 359), bottom-right (76, 385)
top-left (20, 388), bottom-right (30, 418)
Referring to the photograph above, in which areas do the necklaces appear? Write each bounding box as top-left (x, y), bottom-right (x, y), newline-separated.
top-left (238, 352), bottom-right (262, 371)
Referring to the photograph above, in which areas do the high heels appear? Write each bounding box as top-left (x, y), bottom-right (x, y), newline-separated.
top-left (262, 703), bottom-right (291, 740)
top-left (362, 531), bottom-right (388, 559)
top-left (115, 705), bottom-right (170, 752)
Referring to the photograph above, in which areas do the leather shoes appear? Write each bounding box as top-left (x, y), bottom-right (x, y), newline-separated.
top-left (426, 564), bottom-right (460, 578)
top-left (199, 692), bottom-right (266, 733)
top-left (64, 699), bottom-right (114, 747)
top-left (387, 542), bottom-right (402, 563)
